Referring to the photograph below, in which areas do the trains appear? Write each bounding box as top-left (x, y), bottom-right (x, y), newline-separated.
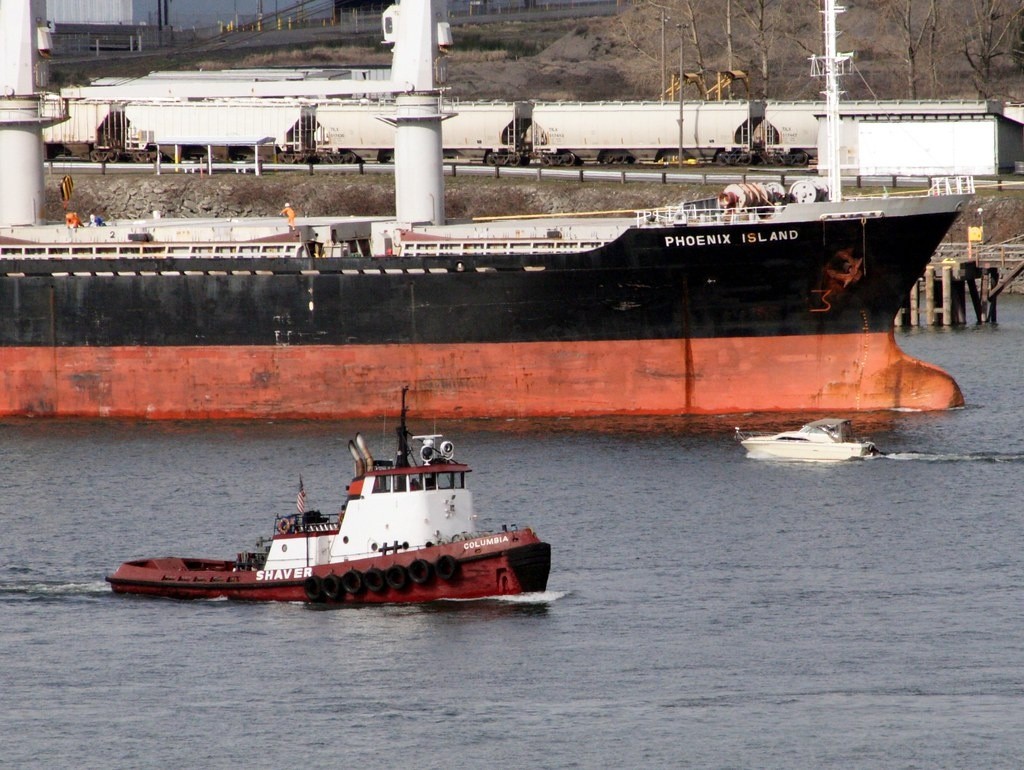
top-left (40, 96), bottom-right (1024, 169)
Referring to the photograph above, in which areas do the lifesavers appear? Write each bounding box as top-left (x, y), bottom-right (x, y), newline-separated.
top-left (322, 574), bottom-right (341, 596)
top-left (434, 555), bottom-right (460, 581)
top-left (303, 574), bottom-right (323, 599)
top-left (341, 569), bottom-right (363, 594)
top-left (338, 510), bottom-right (345, 523)
top-left (388, 564), bottom-right (408, 590)
top-left (279, 518), bottom-right (290, 534)
top-left (408, 558), bottom-right (433, 584)
top-left (362, 567), bottom-right (386, 593)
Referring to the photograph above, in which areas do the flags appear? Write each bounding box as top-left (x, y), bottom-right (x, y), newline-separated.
top-left (295, 475), bottom-right (307, 514)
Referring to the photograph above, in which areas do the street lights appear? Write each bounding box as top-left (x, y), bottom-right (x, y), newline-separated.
top-left (676, 23), bottom-right (690, 170)
top-left (655, 9), bottom-right (670, 101)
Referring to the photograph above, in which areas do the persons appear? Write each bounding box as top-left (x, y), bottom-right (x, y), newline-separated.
top-left (88, 212), bottom-right (106, 227)
top-left (64, 211), bottom-right (85, 228)
top-left (279, 201), bottom-right (296, 231)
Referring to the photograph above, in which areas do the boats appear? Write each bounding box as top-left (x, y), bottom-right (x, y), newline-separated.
top-left (0, 182), bottom-right (965, 435)
top-left (105, 386), bottom-right (552, 606)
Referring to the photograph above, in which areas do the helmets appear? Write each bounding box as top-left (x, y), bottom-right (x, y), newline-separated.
top-left (90, 214), bottom-right (95, 222)
top-left (285, 203), bottom-right (290, 207)
